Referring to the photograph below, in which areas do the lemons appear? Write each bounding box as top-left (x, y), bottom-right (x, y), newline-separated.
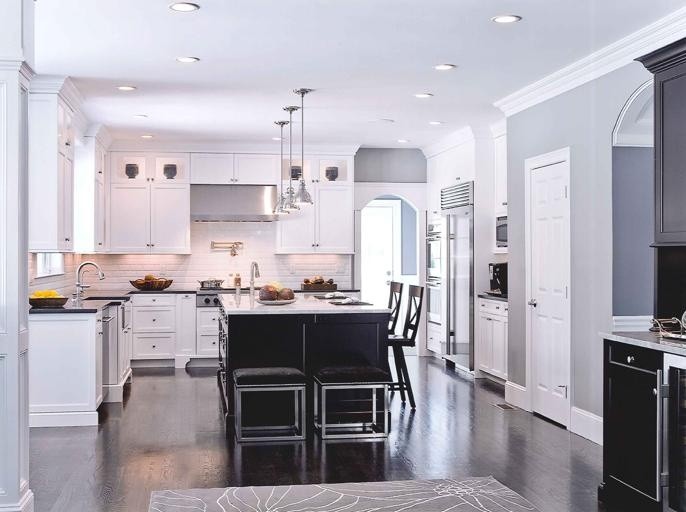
top-left (31, 290), bottom-right (65, 298)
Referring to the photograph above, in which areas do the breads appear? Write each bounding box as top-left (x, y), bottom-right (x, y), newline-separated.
top-left (278, 288), bottom-right (294, 301)
top-left (259, 286), bottom-right (280, 301)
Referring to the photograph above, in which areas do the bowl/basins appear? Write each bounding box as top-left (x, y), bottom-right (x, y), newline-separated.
top-left (129, 280), bottom-right (173, 291)
top-left (28, 296), bottom-right (69, 308)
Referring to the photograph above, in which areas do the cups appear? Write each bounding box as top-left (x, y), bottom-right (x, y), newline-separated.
top-left (163, 164), bottom-right (176, 180)
top-left (289, 165), bottom-right (302, 180)
top-left (325, 167), bottom-right (338, 181)
top-left (125, 164), bottom-right (139, 178)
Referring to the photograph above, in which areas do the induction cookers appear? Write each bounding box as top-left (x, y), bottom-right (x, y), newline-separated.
top-left (195, 288), bottom-right (261, 308)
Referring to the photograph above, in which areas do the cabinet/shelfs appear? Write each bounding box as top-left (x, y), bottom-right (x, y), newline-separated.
top-left (597, 338), bottom-right (663, 511)
top-left (494, 135), bottom-right (508, 217)
top-left (276, 153), bottom-right (355, 255)
top-left (190, 153), bottom-right (277, 185)
top-left (104, 152), bottom-right (190, 255)
top-left (480, 298), bottom-right (507, 387)
top-left (74, 137), bottom-right (104, 255)
top-left (102, 325), bottom-right (132, 403)
top-left (132, 295), bottom-right (196, 368)
top-left (426, 322), bottom-right (441, 354)
top-left (650, 63), bottom-right (686, 248)
top-left (28, 93), bottom-right (74, 252)
top-left (196, 306), bottom-right (219, 367)
top-left (28, 314), bottom-right (102, 428)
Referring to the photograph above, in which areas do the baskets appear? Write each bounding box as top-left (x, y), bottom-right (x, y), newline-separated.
top-left (130, 278), bottom-right (173, 290)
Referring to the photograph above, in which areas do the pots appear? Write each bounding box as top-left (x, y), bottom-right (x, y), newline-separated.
top-left (198, 280), bottom-right (225, 288)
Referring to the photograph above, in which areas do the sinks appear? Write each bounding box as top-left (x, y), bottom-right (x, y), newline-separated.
top-left (87, 299), bottom-right (126, 302)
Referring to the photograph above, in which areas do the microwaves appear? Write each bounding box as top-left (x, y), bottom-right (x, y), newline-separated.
top-left (495, 216), bottom-right (508, 248)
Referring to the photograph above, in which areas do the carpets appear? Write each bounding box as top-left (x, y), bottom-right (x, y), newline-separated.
top-left (149, 475), bottom-right (541, 512)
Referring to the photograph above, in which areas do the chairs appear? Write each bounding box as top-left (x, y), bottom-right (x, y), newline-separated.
top-left (388, 285), bottom-right (424, 412)
top-left (388, 281), bottom-right (403, 394)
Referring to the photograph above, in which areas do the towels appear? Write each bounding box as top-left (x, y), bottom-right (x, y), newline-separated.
top-left (325, 292), bottom-right (346, 297)
top-left (342, 296), bottom-right (359, 304)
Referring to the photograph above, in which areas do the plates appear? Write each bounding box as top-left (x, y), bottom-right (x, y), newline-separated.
top-left (254, 298), bottom-right (296, 306)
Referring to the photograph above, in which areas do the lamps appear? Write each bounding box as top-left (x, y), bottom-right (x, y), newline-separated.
top-left (282, 106), bottom-right (301, 211)
top-left (293, 88), bottom-right (313, 205)
top-left (274, 121), bottom-right (288, 214)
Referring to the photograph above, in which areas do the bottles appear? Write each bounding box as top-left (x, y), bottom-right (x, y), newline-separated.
top-left (227, 273), bottom-right (234, 287)
top-left (234, 273), bottom-right (241, 288)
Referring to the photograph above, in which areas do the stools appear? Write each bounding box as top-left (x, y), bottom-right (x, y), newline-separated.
top-left (314, 368), bottom-right (391, 439)
top-left (233, 367), bottom-right (307, 443)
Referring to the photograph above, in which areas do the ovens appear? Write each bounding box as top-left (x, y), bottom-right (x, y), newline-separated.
top-left (423, 237), bottom-right (444, 325)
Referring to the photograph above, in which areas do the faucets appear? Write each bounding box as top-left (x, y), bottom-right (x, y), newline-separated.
top-left (75, 261), bottom-right (105, 285)
top-left (250, 261), bottom-right (260, 293)
top-left (81, 270), bottom-right (89, 291)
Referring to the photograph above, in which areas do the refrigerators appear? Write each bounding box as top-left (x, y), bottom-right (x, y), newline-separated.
top-left (433, 178), bottom-right (475, 373)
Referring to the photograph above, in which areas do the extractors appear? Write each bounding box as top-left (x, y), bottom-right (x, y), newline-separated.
top-left (190, 184), bottom-right (280, 223)
